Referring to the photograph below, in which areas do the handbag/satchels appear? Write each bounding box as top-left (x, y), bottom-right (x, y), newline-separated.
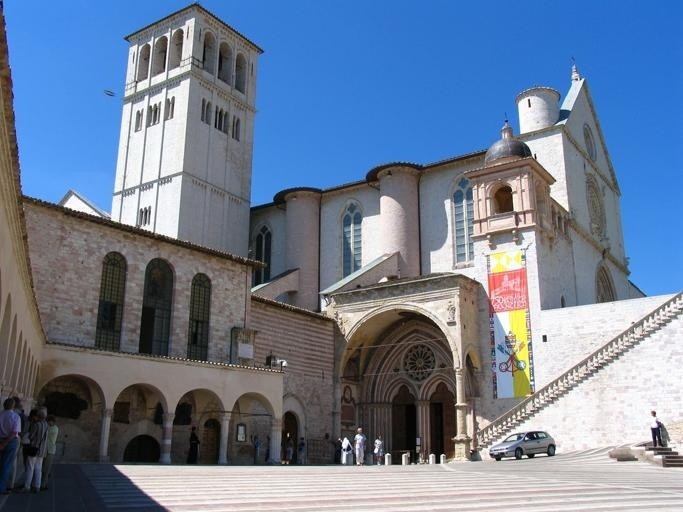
top-left (25, 444), bottom-right (38, 457)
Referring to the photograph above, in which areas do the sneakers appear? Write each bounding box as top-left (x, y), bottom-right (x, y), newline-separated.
top-left (1, 485), bottom-right (48, 494)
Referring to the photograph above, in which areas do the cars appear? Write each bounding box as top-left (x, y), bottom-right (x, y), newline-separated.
top-left (489, 431), bottom-right (556, 461)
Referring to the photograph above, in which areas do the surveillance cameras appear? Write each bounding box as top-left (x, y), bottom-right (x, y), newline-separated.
top-left (282, 361), bottom-right (288, 368)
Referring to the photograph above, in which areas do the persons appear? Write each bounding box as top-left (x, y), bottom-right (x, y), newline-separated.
top-left (353, 427), bottom-right (367, 465)
top-left (286, 437), bottom-right (294, 462)
top-left (0, 395), bottom-right (59, 495)
top-left (250, 433), bottom-right (264, 463)
top-left (649, 410), bottom-right (664, 447)
top-left (187, 426), bottom-right (200, 463)
top-left (335, 437), bottom-right (343, 464)
top-left (342, 435), bottom-right (354, 464)
top-left (298, 436), bottom-right (306, 464)
top-left (373, 431), bottom-right (386, 465)
